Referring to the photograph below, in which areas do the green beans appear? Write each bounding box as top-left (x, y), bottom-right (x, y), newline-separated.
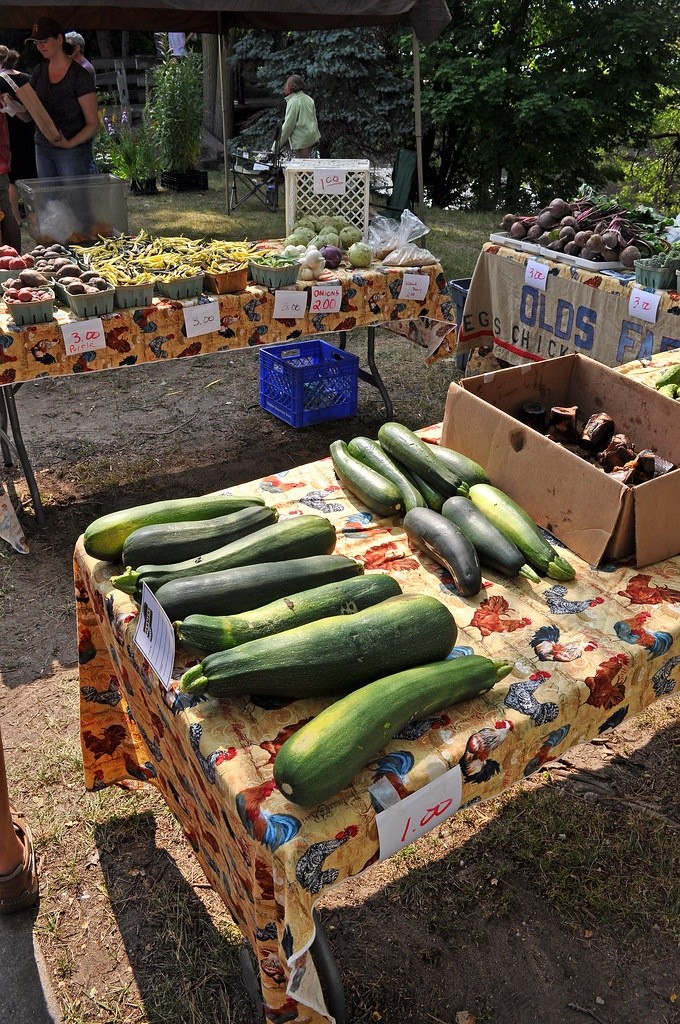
top-left (70, 227), bottom-right (301, 287)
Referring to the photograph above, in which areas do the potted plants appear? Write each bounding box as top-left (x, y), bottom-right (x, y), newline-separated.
top-left (143, 31), bottom-right (209, 191)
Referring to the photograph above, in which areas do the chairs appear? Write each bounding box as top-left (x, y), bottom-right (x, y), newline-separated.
top-left (342, 147), bottom-right (417, 232)
top-left (229, 125), bottom-right (279, 213)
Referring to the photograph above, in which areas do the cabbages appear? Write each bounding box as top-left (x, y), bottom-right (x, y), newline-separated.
top-left (284, 214), bottom-right (372, 268)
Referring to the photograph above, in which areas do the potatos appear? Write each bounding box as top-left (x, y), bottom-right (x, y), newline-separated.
top-left (0, 244), bottom-right (108, 303)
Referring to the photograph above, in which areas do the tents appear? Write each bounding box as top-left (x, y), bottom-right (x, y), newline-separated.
top-left (0, 0), bottom-right (453, 249)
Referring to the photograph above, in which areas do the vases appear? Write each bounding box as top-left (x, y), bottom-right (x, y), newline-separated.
top-left (131, 178), bottom-right (159, 195)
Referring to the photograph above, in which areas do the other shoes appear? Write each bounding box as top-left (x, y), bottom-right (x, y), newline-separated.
top-left (0, 813), bottom-right (38, 916)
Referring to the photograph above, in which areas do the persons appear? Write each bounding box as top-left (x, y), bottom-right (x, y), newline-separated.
top-left (154, 32), bottom-right (194, 54)
top-left (0, 16), bottom-right (99, 256)
top-left (0, 728), bottom-right (39, 912)
top-left (278, 75), bottom-right (322, 210)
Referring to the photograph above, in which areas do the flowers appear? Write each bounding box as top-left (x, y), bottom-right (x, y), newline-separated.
top-left (96, 108), bottom-right (154, 183)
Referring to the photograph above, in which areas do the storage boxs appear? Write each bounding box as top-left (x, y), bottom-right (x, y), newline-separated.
top-left (15, 174), bottom-right (130, 247)
top-left (437, 352), bottom-right (680, 567)
top-left (259, 338), bottom-right (358, 428)
top-left (0, 259), bottom-right (302, 325)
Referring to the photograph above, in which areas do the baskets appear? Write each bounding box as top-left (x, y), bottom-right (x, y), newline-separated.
top-left (634, 259), bottom-right (677, 289)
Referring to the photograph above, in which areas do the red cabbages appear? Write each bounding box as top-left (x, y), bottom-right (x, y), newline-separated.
top-left (318, 245), bottom-right (342, 266)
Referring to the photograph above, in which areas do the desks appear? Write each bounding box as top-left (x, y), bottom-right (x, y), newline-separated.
top-left (0, 239), bottom-right (459, 529)
top-left (76, 420), bottom-right (680, 1024)
top-left (455, 242), bottom-right (679, 366)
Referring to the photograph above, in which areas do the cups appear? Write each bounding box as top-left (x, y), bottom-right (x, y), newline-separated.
top-left (266, 185), bottom-right (275, 206)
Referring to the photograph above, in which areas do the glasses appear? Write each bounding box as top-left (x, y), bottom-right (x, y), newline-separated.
top-left (32, 37), bottom-right (49, 44)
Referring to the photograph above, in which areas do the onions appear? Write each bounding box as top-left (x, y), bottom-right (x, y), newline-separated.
top-left (281, 243), bottom-right (326, 281)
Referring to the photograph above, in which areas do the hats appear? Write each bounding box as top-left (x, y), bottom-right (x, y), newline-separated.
top-left (24, 17), bottom-right (61, 43)
